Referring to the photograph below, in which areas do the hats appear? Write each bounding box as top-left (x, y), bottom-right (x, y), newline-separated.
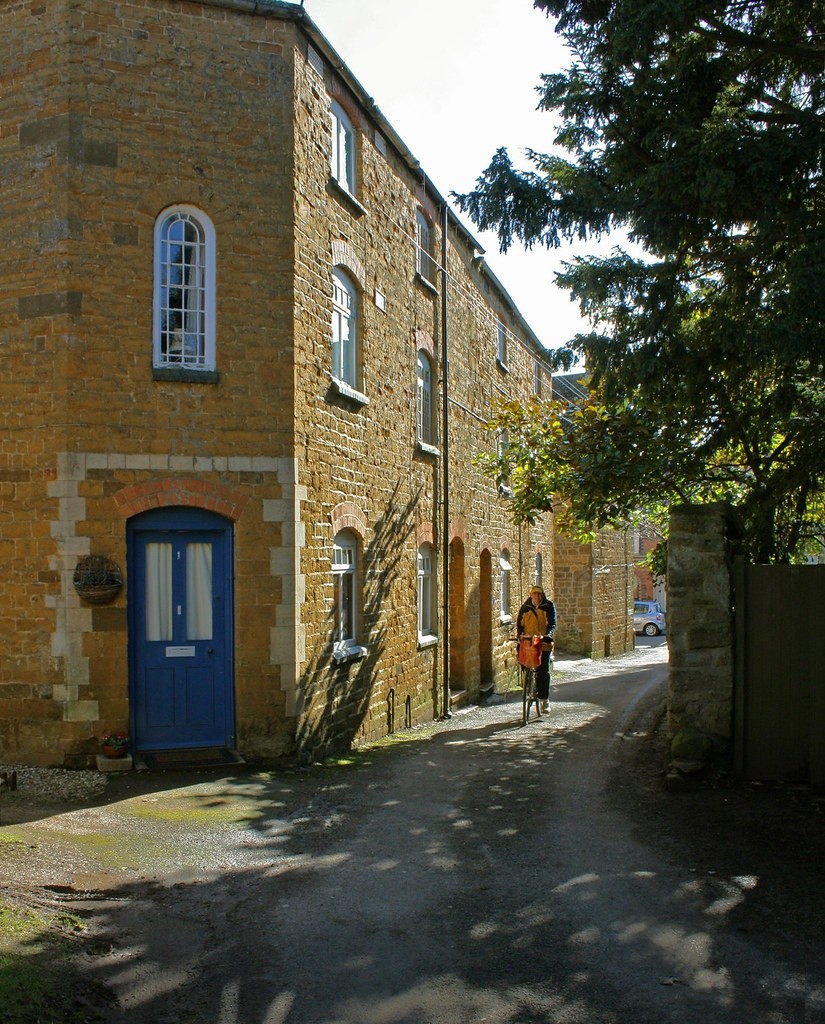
top-left (531, 586), bottom-right (543, 593)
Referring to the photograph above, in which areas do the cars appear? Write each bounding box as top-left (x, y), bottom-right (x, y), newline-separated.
top-left (633, 601), bottom-right (666, 637)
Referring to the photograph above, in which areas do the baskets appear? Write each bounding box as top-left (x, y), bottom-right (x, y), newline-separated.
top-left (520, 642), bottom-right (542, 668)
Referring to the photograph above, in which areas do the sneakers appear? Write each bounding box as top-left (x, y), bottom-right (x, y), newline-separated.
top-left (540, 699), bottom-right (550, 714)
top-left (522, 672), bottom-right (526, 688)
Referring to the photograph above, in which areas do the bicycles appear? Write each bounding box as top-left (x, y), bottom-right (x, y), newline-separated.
top-left (516, 636), bottom-right (554, 725)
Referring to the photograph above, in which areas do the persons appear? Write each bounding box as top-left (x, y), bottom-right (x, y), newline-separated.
top-left (516, 586), bottom-right (556, 713)
